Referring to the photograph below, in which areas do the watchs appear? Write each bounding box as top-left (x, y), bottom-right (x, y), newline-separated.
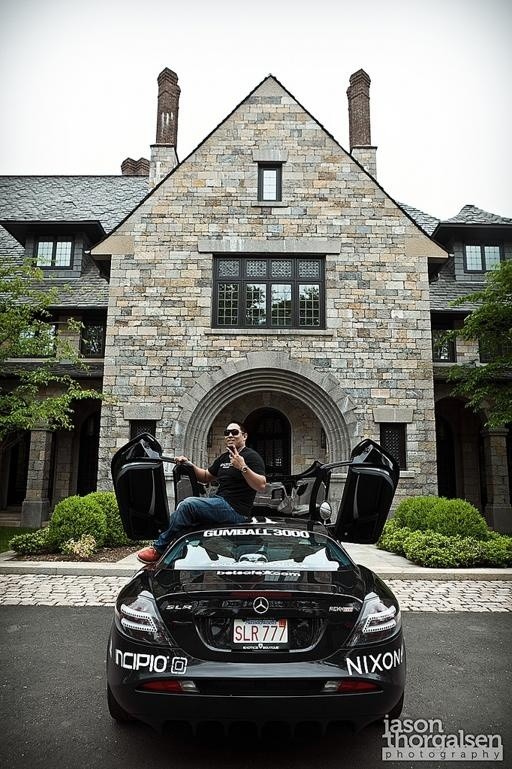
top-left (240, 465), bottom-right (248, 475)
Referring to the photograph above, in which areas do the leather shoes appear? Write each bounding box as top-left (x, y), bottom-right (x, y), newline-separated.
top-left (136, 547), bottom-right (162, 564)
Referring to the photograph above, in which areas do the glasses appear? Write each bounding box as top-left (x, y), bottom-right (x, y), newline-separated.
top-left (224, 429), bottom-right (245, 436)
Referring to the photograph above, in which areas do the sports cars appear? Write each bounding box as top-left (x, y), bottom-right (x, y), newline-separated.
top-left (100, 426), bottom-right (413, 727)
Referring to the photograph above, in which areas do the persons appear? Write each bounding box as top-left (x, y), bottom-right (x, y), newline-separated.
top-left (137, 421), bottom-right (266, 564)
